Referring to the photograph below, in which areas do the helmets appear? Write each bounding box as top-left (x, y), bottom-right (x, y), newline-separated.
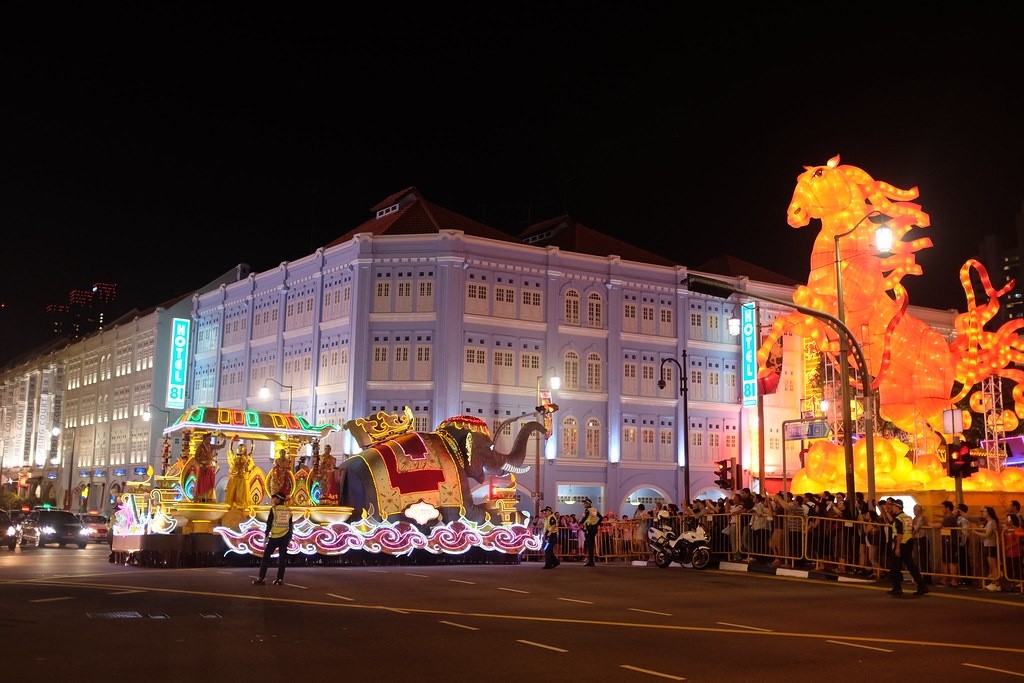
top-left (658, 510), bottom-right (670, 521)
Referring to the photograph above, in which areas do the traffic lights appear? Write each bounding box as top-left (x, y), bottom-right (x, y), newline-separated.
top-left (961, 441), bottom-right (979, 478)
top-left (535, 403), bottom-right (559, 415)
top-left (713, 460), bottom-right (728, 491)
top-left (727, 464), bottom-right (743, 490)
top-left (946, 443), bottom-right (969, 476)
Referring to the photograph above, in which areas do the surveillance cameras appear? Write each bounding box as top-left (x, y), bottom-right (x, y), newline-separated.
top-left (657, 380), bottom-right (666, 389)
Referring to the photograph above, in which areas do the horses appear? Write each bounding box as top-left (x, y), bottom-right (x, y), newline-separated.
top-left (754, 155), bottom-right (1024, 462)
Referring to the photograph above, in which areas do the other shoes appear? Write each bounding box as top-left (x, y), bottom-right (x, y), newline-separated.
top-left (584, 561), bottom-right (595, 566)
top-left (552, 562), bottom-right (560, 568)
top-left (887, 590), bottom-right (903, 595)
top-left (251, 579), bottom-right (265, 584)
top-left (542, 567), bottom-right (550, 569)
top-left (913, 587), bottom-right (929, 596)
top-left (272, 581), bottom-right (282, 585)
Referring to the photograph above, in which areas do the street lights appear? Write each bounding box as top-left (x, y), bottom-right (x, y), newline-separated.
top-left (656, 351), bottom-right (689, 508)
top-left (143, 405), bottom-right (170, 442)
top-left (728, 300), bottom-right (766, 497)
top-left (535, 366), bottom-right (560, 519)
top-left (834, 210), bottom-right (894, 516)
top-left (52, 421), bottom-right (76, 508)
top-left (259, 378), bottom-right (293, 414)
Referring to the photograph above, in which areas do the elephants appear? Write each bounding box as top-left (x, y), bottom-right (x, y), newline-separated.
top-left (336, 415), bottom-right (548, 532)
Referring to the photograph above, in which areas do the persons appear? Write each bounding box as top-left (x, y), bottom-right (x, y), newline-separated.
top-left (555, 512), bottom-right (585, 563)
top-left (927, 500), bottom-right (1024, 593)
top-left (224, 435), bottom-right (254, 509)
top-left (541, 507), bottom-right (560, 569)
top-left (318, 445), bottom-right (338, 506)
top-left (251, 492), bottom-right (293, 586)
top-left (529, 516), bottom-right (540, 533)
top-left (193, 433), bottom-right (227, 504)
top-left (886, 499), bottom-right (929, 596)
top-left (912, 503), bottom-right (933, 584)
top-left (600, 488), bottom-right (895, 582)
top-left (271, 448), bottom-right (292, 496)
top-left (578, 498), bottom-right (603, 567)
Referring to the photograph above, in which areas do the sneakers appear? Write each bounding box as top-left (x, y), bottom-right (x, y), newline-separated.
top-left (985, 583), bottom-right (995, 589)
top-left (987, 585), bottom-right (1002, 593)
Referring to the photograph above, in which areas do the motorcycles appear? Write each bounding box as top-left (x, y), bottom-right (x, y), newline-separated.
top-left (647, 510), bottom-right (712, 570)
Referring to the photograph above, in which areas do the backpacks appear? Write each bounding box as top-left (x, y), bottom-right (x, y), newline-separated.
top-left (804, 503), bottom-right (816, 522)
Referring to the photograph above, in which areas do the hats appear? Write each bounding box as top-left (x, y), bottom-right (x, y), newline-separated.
top-left (892, 500), bottom-right (904, 509)
top-left (271, 492), bottom-right (286, 501)
top-left (582, 499), bottom-right (592, 505)
top-left (541, 506), bottom-right (552, 512)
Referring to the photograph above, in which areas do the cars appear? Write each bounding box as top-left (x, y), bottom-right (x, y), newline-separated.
top-left (0, 504), bottom-right (110, 551)
